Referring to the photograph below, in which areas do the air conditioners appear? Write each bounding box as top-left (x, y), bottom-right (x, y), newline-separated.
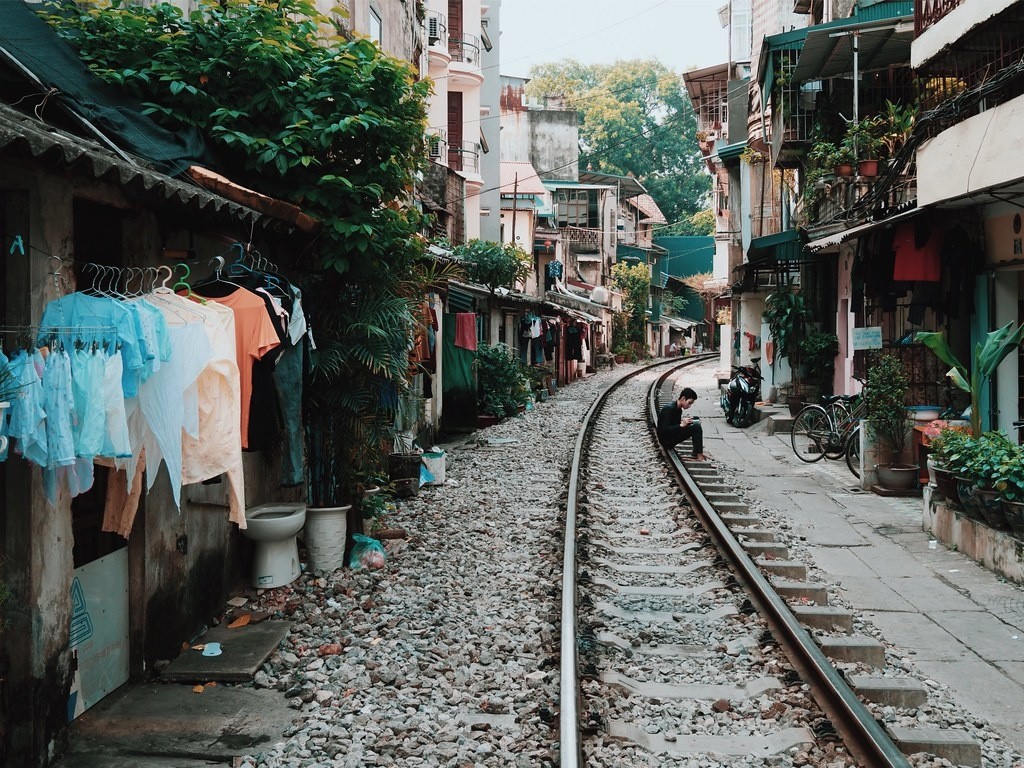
top-left (424, 16), bottom-right (441, 45)
top-left (428, 135), bottom-right (440, 157)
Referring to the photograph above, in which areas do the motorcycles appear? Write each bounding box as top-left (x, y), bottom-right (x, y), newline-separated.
top-left (720, 365), bottom-right (765, 428)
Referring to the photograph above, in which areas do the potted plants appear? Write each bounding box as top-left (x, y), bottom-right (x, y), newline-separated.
top-left (822, 153), bottom-right (835, 183)
top-left (810, 141), bottom-right (835, 188)
top-left (883, 99), bottom-right (918, 176)
top-left (308, 258), bottom-right (454, 574)
top-left (475, 343), bottom-right (559, 424)
top-left (973, 429), bottom-right (1013, 532)
top-left (825, 147), bottom-right (856, 178)
top-left (762, 277), bottom-right (813, 418)
top-left (993, 443), bottom-right (1024, 541)
top-left (861, 348), bottom-right (920, 491)
top-left (856, 117), bottom-right (886, 177)
top-left (944, 434), bottom-right (979, 518)
top-left (931, 430), bottom-right (970, 502)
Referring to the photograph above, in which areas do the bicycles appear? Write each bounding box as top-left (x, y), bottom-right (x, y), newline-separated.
top-left (790, 374), bottom-right (869, 480)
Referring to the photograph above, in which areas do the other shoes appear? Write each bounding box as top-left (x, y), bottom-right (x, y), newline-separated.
top-left (691, 448), bottom-right (706, 460)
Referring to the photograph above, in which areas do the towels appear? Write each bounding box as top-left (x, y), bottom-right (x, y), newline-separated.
top-left (766, 341), bottom-right (777, 365)
top-left (455, 313), bottom-right (479, 350)
top-left (749, 335), bottom-right (760, 350)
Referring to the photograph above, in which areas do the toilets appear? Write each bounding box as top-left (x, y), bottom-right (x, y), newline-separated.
top-left (236, 501), bottom-right (306, 588)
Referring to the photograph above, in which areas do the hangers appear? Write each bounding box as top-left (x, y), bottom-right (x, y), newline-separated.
top-left (81, 246), bottom-right (294, 302)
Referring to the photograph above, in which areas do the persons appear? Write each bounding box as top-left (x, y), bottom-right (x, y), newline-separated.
top-left (669, 335), bottom-right (687, 357)
top-left (656, 387), bottom-right (706, 462)
